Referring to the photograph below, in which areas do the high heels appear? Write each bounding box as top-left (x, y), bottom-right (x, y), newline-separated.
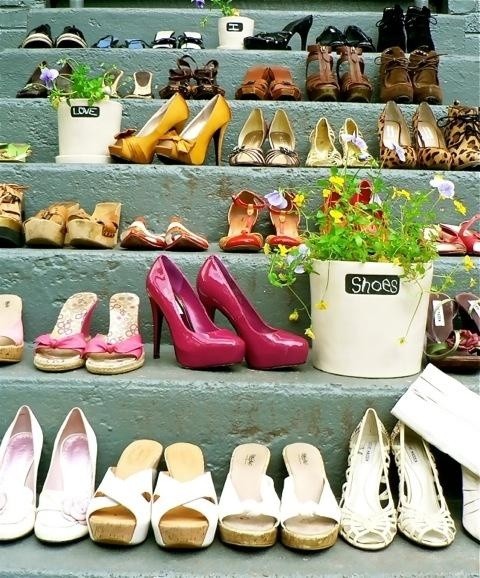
top-left (144, 252), bottom-right (245, 371)
top-left (21, 5), bottom-right (437, 54)
top-left (108, 92), bottom-right (480, 172)
top-left (196, 254), bottom-right (309, 369)
top-left (0, 183), bottom-right (479, 257)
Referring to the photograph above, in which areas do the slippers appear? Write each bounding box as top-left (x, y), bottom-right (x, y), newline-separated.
top-left (424, 292), bottom-right (480, 373)
top-left (34, 292), bottom-right (97, 373)
top-left (0, 293), bottom-right (25, 363)
top-left (82, 292), bottom-right (145, 374)
top-left (456, 291), bottom-right (480, 334)
top-left (86, 438), bottom-right (340, 550)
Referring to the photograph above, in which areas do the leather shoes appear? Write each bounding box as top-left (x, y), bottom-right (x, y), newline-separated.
top-left (34, 406), bottom-right (97, 542)
top-left (339, 408), bottom-right (480, 549)
top-left (0, 403), bottom-right (44, 542)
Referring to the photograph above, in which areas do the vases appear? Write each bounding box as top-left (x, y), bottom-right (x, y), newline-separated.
top-left (310, 258), bottom-right (433, 380)
top-left (216, 16), bottom-right (255, 48)
top-left (56, 98), bottom-right (123, 163)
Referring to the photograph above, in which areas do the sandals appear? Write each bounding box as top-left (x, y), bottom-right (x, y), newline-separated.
top-left (16, 46), bottom-right (443, 98)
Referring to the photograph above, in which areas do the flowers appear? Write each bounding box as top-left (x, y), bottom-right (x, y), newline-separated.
top-left (192, 0), bottom-right (241, 24)
top-left (24, 56), bottom-right (121, 105)
top-left (263, 155), bottom-right (476, 342)
